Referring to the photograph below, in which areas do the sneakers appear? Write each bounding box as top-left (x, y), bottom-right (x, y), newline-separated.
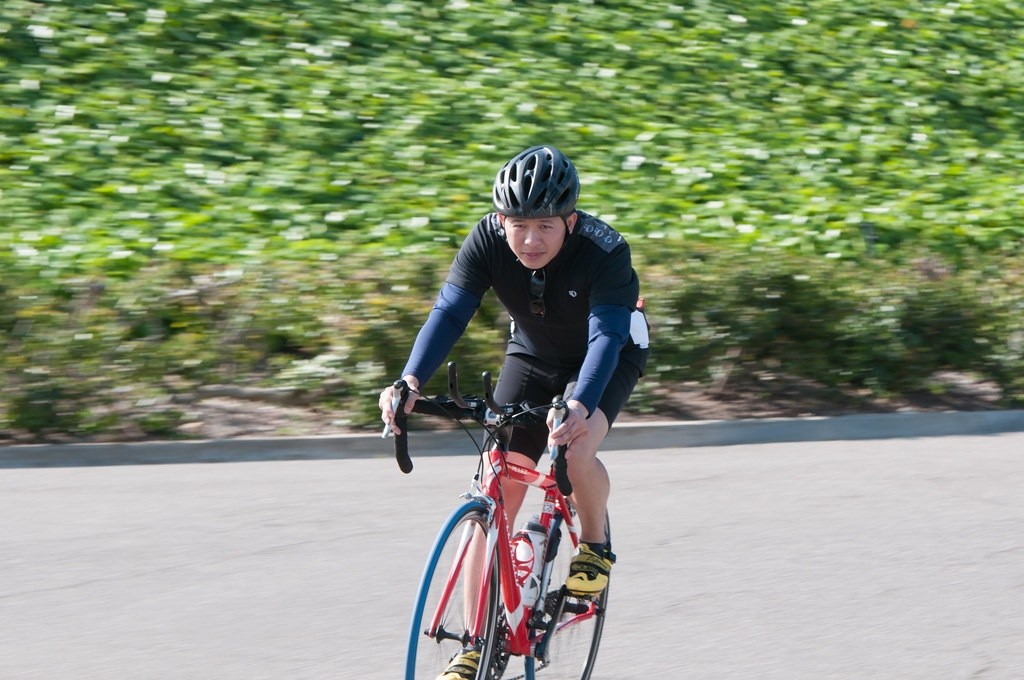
top-left (566, 531), bottom-right (616, 595)
top-left (437, 648), bottom-right (481, 680)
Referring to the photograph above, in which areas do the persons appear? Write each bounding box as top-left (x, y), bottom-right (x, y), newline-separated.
top-left (379, 145), bottom-right (649, 680)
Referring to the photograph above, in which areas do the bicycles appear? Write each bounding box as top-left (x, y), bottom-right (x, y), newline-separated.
top-left (378, 361), bottom-right (616, 680)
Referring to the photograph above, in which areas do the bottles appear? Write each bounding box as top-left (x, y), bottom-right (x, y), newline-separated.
top-left (510, 514), bottom-right (549, 609)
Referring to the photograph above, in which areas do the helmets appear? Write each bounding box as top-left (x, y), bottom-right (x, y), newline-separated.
top-left (492, 145), bottom-right (581, 219)
